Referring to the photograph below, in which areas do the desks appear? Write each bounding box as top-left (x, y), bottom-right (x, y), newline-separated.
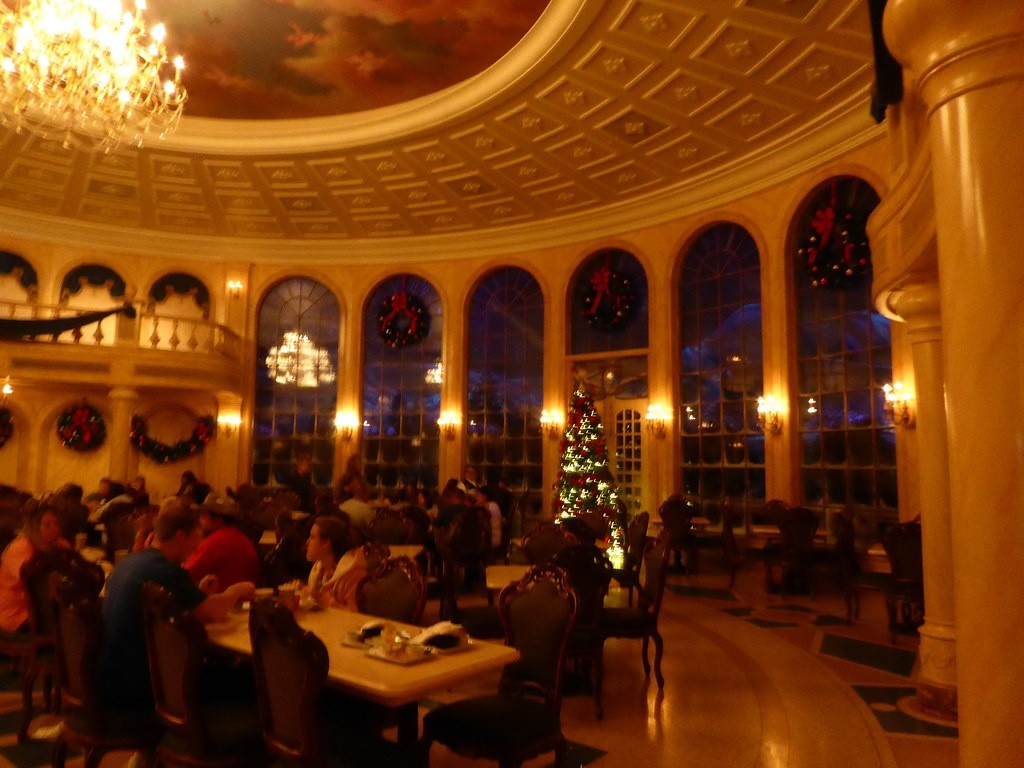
top-left (387, 544), bottom-right (424, 561)
top-left (486, 566), bottom-right (620, 609)
top-left (259, 532), bottom-right (276, 556)
top-left (206, 588), bottom-right (520, 768)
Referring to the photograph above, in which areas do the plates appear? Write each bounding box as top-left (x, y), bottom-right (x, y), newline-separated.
top-left (340, 634), bottom-right (478, 665)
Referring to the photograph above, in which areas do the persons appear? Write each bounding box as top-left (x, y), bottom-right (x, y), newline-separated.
top-left (0, 457), bottom-right (513, 732)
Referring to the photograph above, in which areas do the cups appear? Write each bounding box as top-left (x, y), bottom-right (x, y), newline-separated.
top-left (75, 533), bottom-right (87, 552)
top-left (115, 550), bottom-right (129, 567)
top-left (254, 588), bottom-right (273, 601)
top-left (292, 511), bottom-right (310, 520)
top-left (278, 585), bottom-right (295, 611)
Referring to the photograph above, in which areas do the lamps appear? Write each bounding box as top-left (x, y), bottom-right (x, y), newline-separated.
top-left (228, 281), bottom-right (242, 299)
top-left (539, 409), bottom-right (560, 439)
top-left (883, 384), bottom-right (915, 433)
top-left (0, 0), bottom-right (189, 154)
top-left (645, 403), bottom-right (669, 438)
top-left (218, 414), bottom-right (242, 437)
top-left (2, 384), bottom-right (13, 405)
top-left (334, 410), bottom-right (359, 440)
top-left (757, 397), bottom-right (784, 435)
top-left (437, 410), bottom-right (461, 441)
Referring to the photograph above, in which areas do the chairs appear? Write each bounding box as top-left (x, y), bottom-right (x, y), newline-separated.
top-left (0, 486), bottom-right (924, 768)
